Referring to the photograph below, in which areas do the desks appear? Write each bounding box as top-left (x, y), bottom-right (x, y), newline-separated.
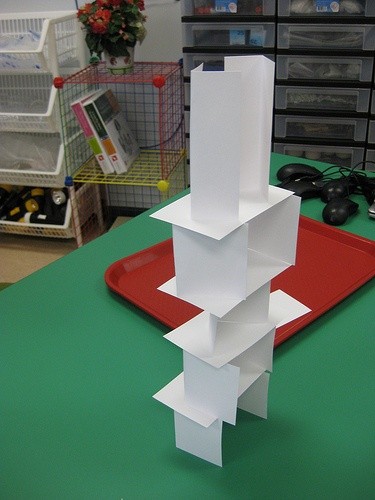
top-left (0, 155), bottom-right (375, 500)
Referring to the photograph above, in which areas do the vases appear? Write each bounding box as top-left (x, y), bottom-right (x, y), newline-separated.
top-left (103, 41), bottom-right (136, 75)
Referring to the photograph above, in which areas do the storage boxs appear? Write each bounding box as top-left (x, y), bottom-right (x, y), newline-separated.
top-left (182, 47), bottom-right (275, 77)
top-left (180, 16), bottom-right (276, 46)
top-left (184, 106), bottom-right (191, 185)
top-left (179, 0), bottom-right (275, 15)
top-left (273, 0), bottom-right (375, 170)
top-left (183, 78), bottom-right (191, 106)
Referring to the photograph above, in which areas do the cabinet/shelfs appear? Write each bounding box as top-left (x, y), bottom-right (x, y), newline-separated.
top-left (0, 8), bottom-right (103, 247)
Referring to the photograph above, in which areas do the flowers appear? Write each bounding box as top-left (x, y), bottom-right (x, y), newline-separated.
top-left (77, 0), bottom-right (149, 57)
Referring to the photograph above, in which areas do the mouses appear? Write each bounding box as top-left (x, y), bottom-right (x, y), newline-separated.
top-left (281, 181), bottom-right (322, 200)
top-left (322, 199), bottom-right (358, 225)
top-left (321, 175), bottom-right (358, 202)
top-left (278, 161), bottom-right (319, 181)
